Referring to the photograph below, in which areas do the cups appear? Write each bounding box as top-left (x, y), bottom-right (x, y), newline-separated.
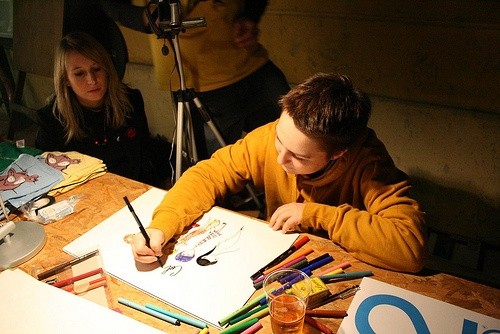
top-left (263, 268), bottom-right (313, 334)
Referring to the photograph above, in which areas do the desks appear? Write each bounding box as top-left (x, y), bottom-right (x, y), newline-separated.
top-left (0, 137), bottom-right (500, 334)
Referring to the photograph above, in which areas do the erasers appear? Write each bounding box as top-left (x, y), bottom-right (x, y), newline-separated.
top-left (291, 277), bottom-right (328, 305)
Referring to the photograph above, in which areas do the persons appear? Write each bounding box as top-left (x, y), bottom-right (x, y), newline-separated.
top-left (35, 35), bottom-right (157, 182)
top-left (131, 73), bottom-right (428, 272)
top-left (110, 0), bottom-right (291, 197)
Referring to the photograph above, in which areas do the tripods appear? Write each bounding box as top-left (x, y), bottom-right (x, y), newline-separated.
top-left (171, 34), bottom-right (265, 212)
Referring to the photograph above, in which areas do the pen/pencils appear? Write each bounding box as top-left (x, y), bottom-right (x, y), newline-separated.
top-left (117, 296), bottom-right (179, 325)
top-left (123, 196), bottom-right (163, 267)
top-left (36, 249), bottom-right (100, 280)
top-left (146, 303), bottom-right (207, 328)
top-left (50, 268), bottom-right (103, 288)
top-left (191, 235), bottom-right (372, 334)
top-left (64, 276), bottom-right (107, 295)
